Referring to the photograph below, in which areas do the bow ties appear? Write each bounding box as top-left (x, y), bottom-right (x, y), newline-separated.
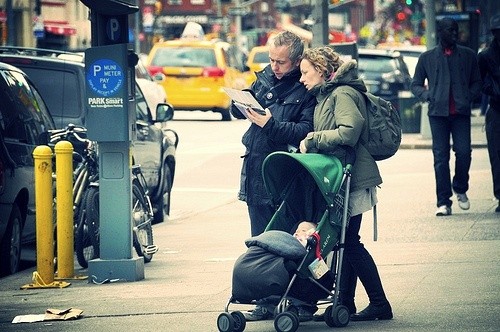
top-left (444, 48), bottom-right (453, 56)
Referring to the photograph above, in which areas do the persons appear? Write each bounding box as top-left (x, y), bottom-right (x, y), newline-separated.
top-left (231, 31), bottom-right (319, 323)
top-left (292, 220), bottom-right (319, 250)
top-left (410, 16), bottom-right (481, 217)
top-left (478, 13), bottom-right (500, 213)
top-left (300, 47), bottom-right (394, 321)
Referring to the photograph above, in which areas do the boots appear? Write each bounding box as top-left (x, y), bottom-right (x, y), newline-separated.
top-left (346, 247), bottom-right (394, 320)
top-left (311, 262), bottom-right (357, 321)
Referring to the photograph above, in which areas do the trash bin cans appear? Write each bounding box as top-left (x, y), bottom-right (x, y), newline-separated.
top-left (399, 97), bottom-right (421, 133)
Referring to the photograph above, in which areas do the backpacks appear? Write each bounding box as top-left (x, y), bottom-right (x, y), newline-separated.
top-left (354, 87), bottom-right (402, 161)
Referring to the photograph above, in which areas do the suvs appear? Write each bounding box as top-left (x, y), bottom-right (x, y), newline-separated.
top-left (0, 45), bottom-right (179, 225)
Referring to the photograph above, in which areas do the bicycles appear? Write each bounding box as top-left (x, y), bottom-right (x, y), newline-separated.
top-left (47, 123), bottom-right (159, 269)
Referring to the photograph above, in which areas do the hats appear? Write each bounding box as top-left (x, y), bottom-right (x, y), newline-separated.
top-left (437, 17), bottom-right (458, 32)
top-left (488, 14), bottom-right (500, 32)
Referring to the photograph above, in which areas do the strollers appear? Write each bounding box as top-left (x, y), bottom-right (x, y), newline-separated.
top-left (216, 144), bottom-right (357, 332)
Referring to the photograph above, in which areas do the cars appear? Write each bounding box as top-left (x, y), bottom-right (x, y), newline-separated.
top-left (357, 45), bottom-right (426, 133)
top-left (58, 51), bottom-right (167, 121)
top-left (0, 62), bottom-right (59, 278)
top-left (146, 21), bottom-right (272, 121)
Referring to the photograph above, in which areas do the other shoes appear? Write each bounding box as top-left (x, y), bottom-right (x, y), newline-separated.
top-left (296, 309), bottom-right (313, 322)
top-left (456, 193), bottom-right (470, 210)
top-left (495, 202), bottom-right (500, 212)
top-left (436, 205), bottom-right (453, 215)
top-left (244, 306), bottom-right (275, 321)
